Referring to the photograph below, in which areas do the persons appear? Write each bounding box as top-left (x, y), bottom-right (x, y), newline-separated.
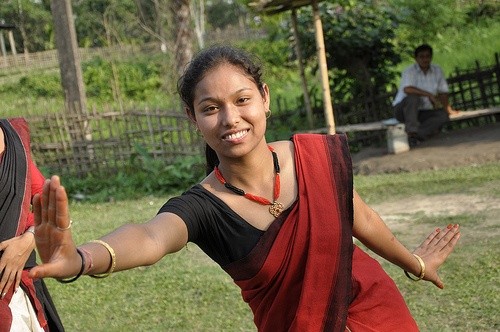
top-left (29, 42), bottom-right (461, 332)
top-left (389, 44), bottom-right (450, 147)
top-left (0, 116), bottom-right (65, 332)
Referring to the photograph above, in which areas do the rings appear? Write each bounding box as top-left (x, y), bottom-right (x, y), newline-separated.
top-left (56, 219), bottom-right (73, 230)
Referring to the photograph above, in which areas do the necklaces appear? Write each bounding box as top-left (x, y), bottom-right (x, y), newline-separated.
top-left (214, 143), bottom-right (285, 218)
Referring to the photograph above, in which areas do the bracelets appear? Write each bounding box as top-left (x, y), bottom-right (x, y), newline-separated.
top-left (404, 253), bottom-right (426, 281)
top-left (84, 239), bottom-right (115, 279)
top-left (25, 228), bottom-right (36, 235)
top-left (55, 246), bottom-right (94, 284)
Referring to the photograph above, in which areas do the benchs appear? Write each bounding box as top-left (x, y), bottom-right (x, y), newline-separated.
top-left (301, 105), bottom-right (500, 154)
